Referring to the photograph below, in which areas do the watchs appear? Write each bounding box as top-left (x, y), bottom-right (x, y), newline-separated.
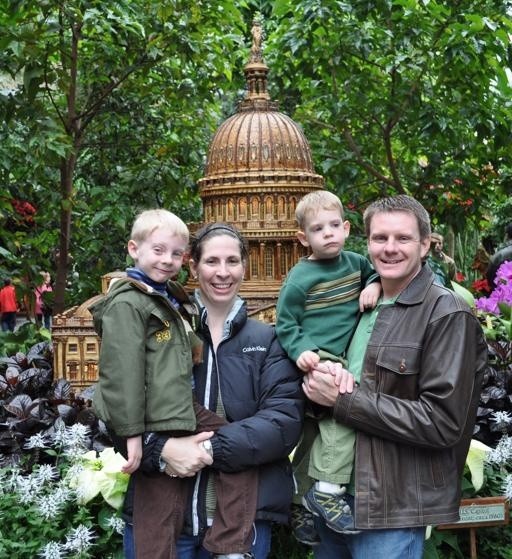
top-left (204, 439), bottom-right (214, 458)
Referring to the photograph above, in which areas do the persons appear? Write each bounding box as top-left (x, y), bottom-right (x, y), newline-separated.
top-left (273, 190), bottom-right (383, 547)
top-left (33, 271), bottom-right (54, 330)
top-left (104, 222), bottom-right (305, 558)
top-left (1, 278), bottom-right (23, 333)
top-left (426, 230), bottom-right (458, 291)
top-left (298, 192), bottom-right (488, 558)
top-left (86, 207), bottom-right (259, 557)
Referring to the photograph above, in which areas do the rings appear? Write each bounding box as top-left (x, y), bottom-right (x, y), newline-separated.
top-left (168, 471), bottom-right (177, 479)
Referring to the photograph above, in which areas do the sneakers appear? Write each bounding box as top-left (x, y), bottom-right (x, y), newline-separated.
top-left (210, 552), bottom-right (256, 559)
top-left (289, 481), bottom-right (363, 546)
